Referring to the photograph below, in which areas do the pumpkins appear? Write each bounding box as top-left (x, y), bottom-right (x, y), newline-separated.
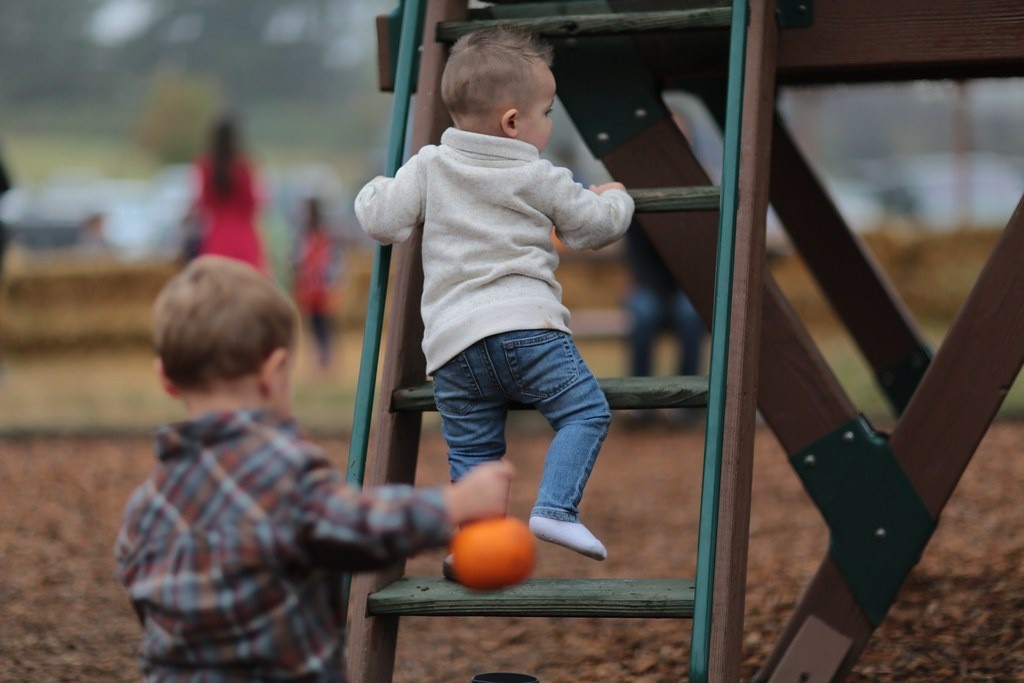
top-left (452, 516), bottom-right (537, 591)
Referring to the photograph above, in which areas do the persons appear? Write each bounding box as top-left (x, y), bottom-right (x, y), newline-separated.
top-left (624, 222), bottom-right (708, 426)
top-left (293, 199), bottom-right (330, 366)
top-left (115, 254), bottom-right (517, 683)
top-left (190, 121), bottom-right (265, 276)
top-left (359, 27), bottom-right (634, 580)
